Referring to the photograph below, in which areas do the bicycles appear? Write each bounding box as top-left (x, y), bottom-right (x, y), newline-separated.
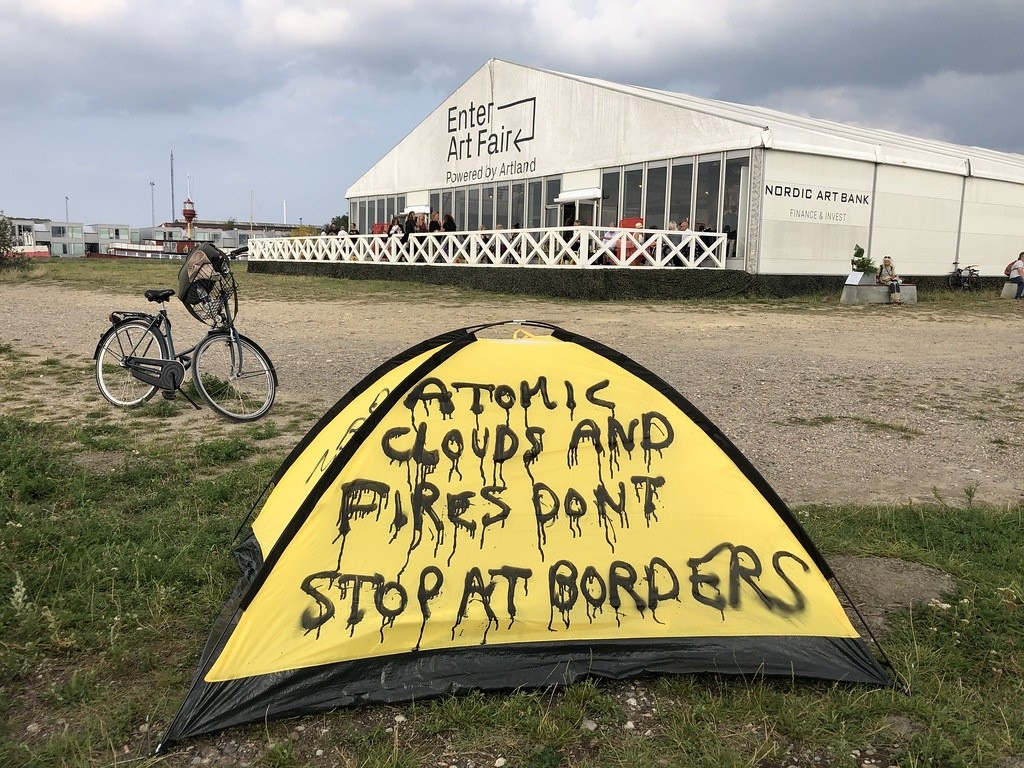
top-left (92, 243), bottom-right (279, 423)
top-left (948, 261), bottom-right (983, 294)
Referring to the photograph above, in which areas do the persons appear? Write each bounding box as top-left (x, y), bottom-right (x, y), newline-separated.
top-left (319, 223), bottom-right (360, 245)
top-left (480, 222), bottom-right (521, 264)
top-left (564, 214), bottom-right (694, 265)
top-left (1009, 252), bottom-right (1024, 299)
top-left (698, 225), bottom-right (737, 254)
top-left (875, 256), bottom-right (905, 304)
top-left (386, 210), bottom-right (459, 260)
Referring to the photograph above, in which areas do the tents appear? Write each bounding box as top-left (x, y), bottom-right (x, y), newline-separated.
top-left (145, 315), bottom-right (915, 760)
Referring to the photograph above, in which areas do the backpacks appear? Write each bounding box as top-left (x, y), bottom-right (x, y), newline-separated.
top-left (1004, 261), bottom-right (1018, 276)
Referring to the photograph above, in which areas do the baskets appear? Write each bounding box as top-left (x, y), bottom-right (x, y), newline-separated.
top-left (969, 270), bottom-right (979, 274)
top-left (182, 263), bottom-right (239, 321)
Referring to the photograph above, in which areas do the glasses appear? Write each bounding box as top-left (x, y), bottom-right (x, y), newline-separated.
top-left (884, 256), bottom-right (891, 259)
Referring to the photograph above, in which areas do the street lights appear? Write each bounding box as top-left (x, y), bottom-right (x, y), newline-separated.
top-left (65, 196), bottom-right (69, 221)
top-left (149, 181), bottom-right (156, 227)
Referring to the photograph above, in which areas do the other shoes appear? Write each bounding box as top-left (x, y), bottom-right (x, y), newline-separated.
top-left (893, 298), bottom-right (904, 305)
top-left (1015, 294), bottom-right (1024, 299)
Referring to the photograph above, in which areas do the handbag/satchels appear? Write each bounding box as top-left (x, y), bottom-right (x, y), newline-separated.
top-left (177, 243), bottom-right (237, 329)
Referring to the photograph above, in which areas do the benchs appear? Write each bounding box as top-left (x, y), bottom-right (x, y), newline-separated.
top-left (1000, 281), bottom-right (1024, 299)
top-left (839, 283), bottom-right (917, 304)
